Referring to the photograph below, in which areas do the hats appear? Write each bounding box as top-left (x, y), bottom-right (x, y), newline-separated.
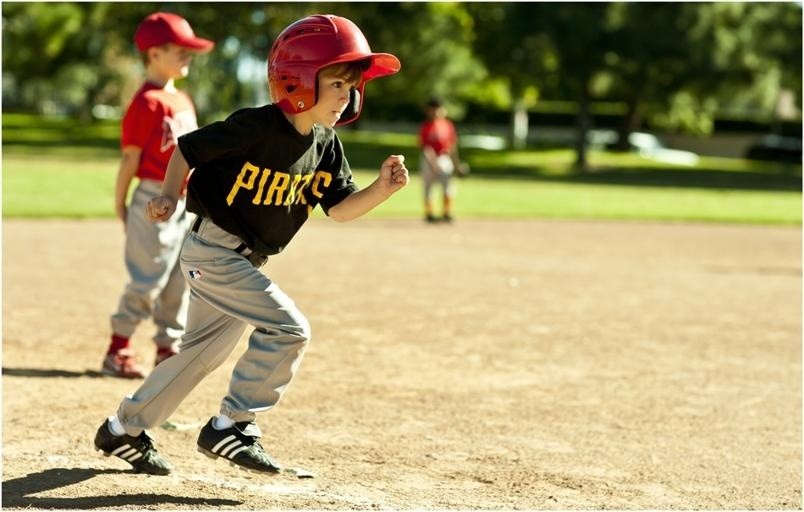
top-left (136, 13), bottom-right (215, 53)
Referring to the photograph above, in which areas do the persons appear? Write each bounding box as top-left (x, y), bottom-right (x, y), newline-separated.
top-left (103, 12), bottom-right (216, 378)
top-left (417, 100), bottom-right (463, 222)
top-left (93, 13), bottom-right (408, 476)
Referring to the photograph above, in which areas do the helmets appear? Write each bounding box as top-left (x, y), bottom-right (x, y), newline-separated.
top-left (267, 14), bottom-right (402, 127)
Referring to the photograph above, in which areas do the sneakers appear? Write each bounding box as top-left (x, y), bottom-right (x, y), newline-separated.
top-left (102, 348), bottom-right (145, 378)
top-left (196, 416), bottom-right (280, 475)
top-left (94, 417), bottom-right (173, 476)
